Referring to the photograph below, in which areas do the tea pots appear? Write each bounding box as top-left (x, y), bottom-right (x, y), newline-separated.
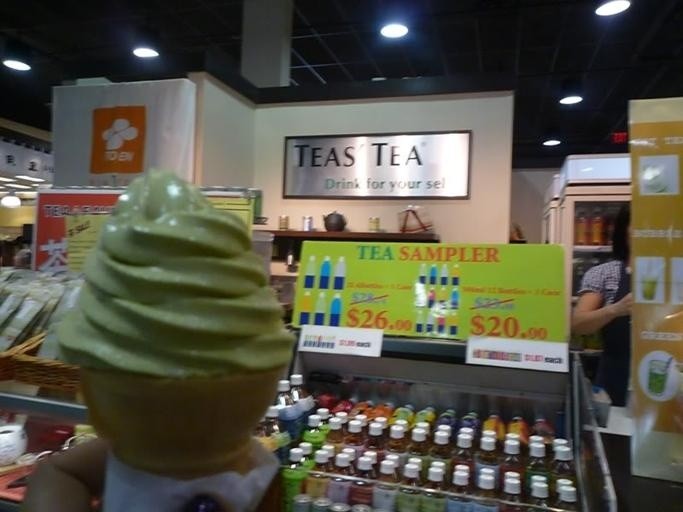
top-left (322, 210), bottom-right (347, 232)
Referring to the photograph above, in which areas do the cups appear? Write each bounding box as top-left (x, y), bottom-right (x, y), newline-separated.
top-left (648, 359), bottom-right (670, 396)
top-left (641, 274), bottom-right (658, 301)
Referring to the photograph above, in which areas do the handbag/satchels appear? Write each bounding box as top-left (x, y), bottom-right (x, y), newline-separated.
top-left (400, 206), bottom-right (433, 234)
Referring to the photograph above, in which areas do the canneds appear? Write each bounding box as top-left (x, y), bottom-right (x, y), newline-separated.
top-left (303, 217), bottom-right (312, 231)
top-left (292, 493), bottom-right (372, 512)
top-left (279, 215), bottom-right (289, 229)
top-left (368, 217), bottom-right (379, 230)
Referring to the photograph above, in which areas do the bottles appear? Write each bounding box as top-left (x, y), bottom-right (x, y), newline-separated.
top-left (414, 262), bottom-right (460, 337)
top-left (572, 207), bottom-right (620, 297)
top-left (250, 372), bottom-right (581, 511)
top-left (300, 254), bottom-right (346, 326)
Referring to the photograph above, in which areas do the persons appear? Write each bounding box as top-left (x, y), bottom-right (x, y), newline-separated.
top-left (17, 436), bottom-right (284, 512)
top-left (572, 201), bottom-right (634, 408)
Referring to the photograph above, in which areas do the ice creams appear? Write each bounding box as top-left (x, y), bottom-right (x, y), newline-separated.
top-left (56, 168), bottom-right (298, 512)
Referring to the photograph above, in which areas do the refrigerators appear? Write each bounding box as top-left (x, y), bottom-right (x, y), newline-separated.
top-left (541, 151), bottom-right (632, 353)
